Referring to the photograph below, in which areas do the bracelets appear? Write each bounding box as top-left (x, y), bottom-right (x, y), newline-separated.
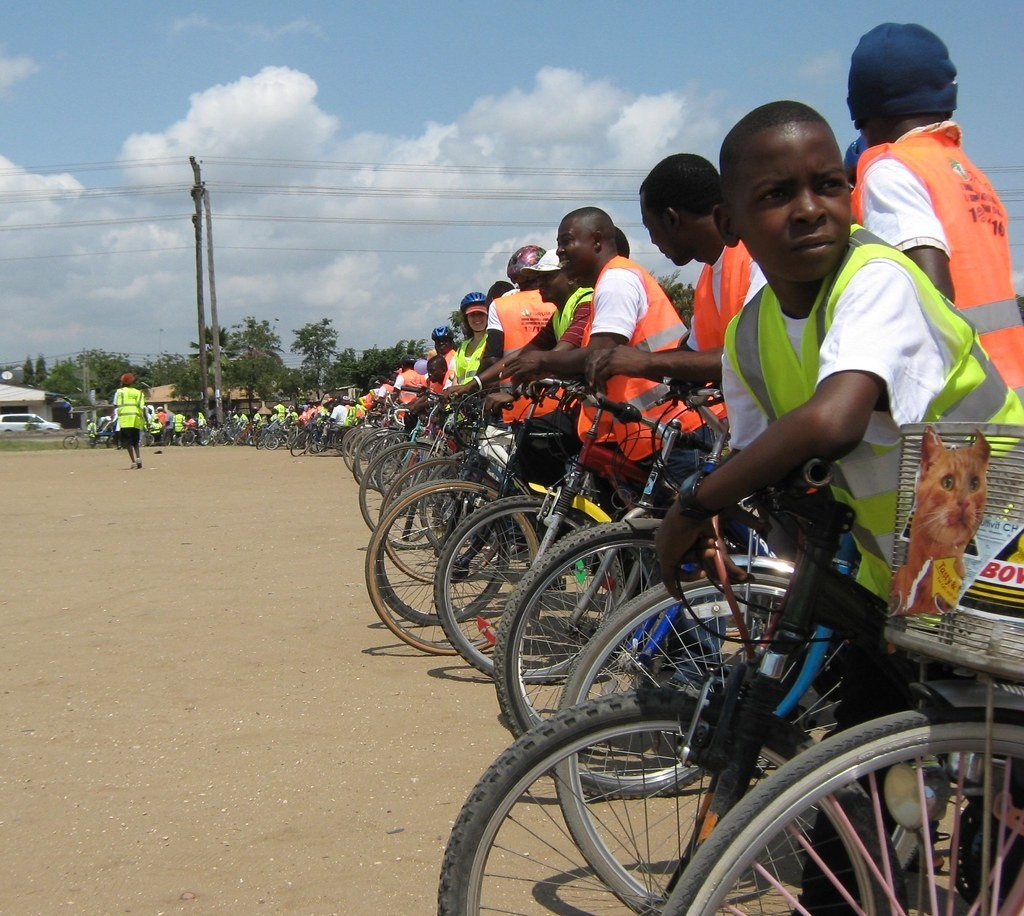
top-left (473, 376), bottom-right (482, 391)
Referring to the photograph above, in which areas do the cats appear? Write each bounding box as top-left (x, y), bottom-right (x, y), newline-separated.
top-left (886, 425), bottom-right (991, 619)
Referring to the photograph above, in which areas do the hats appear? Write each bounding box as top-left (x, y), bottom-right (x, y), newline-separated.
top-left (156, 406), bottom-right (163, 410)
top-left (847, 22), bottom-right (958, 118)
top-left (121, 373), bottom-right (134, 385)
top-left (464, 303), bottom-right (488, 315)
top-left (342, 396), bottom-right (351, 401)
top-left (843, 135), bottom-right (868, 187)
top-left (520, 249), bottom-right (563, 277)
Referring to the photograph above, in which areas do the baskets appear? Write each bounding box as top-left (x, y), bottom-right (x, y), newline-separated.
top-left (884, 421), bottom-right (1024, 680)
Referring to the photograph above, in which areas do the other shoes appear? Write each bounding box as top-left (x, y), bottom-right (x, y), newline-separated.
top-left (131, 462), bottom-right (137, 469)
top-left (136, 458), bottom-right (142, 468)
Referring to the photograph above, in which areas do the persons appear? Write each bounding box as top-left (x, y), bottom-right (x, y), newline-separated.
top-left (83, 22), bottom-right (1024, 601)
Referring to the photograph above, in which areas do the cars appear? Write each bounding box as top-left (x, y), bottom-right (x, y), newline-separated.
top-left (0, 413), bottom-right (62, 432)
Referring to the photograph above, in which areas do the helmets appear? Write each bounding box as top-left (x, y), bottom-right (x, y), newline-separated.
top-left (432, 326), bottom-right (454, 340)
top-left (507, 245), bottom-right (547, 283)
top-left (460, 292), bottom-right (486, 311)
top-left (253, 407), bottom-right (259, 411)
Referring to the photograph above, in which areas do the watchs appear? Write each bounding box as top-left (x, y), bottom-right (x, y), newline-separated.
top-left (679, 470), bottom-right (726, 520)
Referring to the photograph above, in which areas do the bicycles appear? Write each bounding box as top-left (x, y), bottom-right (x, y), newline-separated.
top-left (59, 376), bottom-right (1024, 915)
